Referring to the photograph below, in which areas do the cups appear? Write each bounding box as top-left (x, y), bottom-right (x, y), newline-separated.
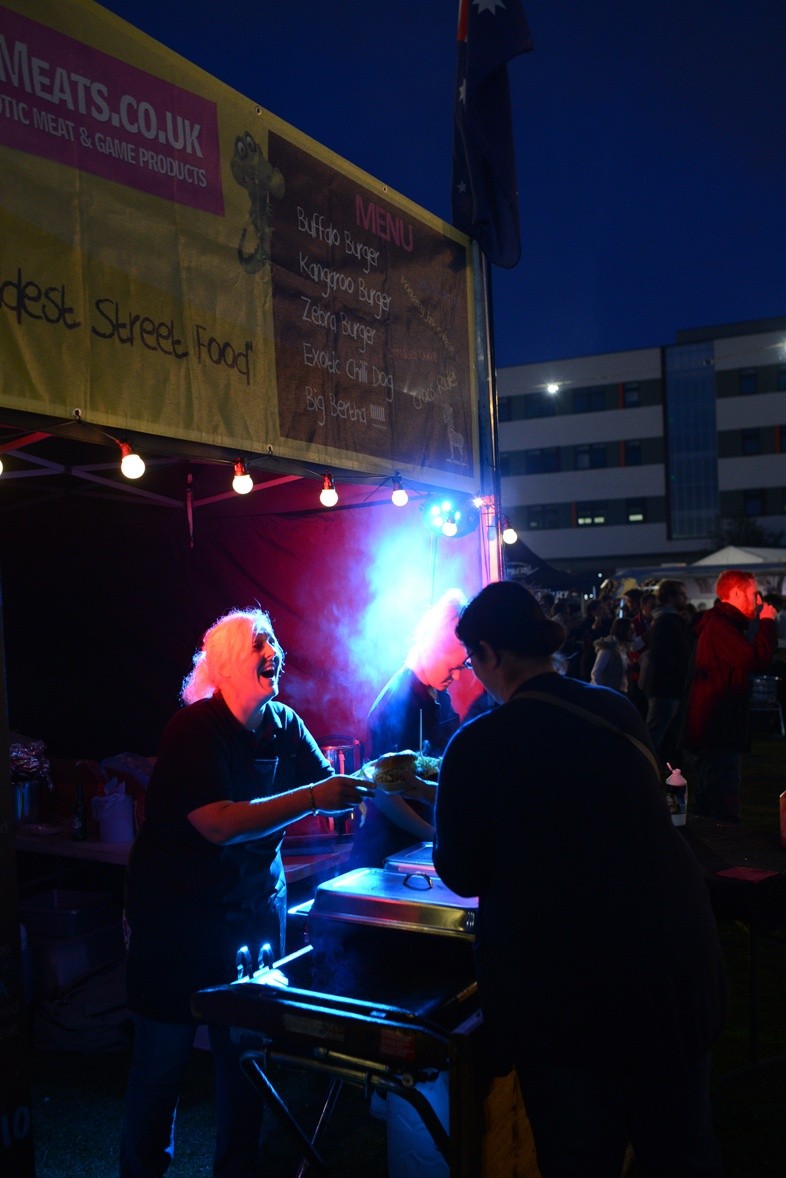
top-left (99, 798), bottom-right (133, 843)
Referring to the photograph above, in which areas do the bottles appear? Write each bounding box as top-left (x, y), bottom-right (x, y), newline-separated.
top-left (23, 777), bottom-right (32, 824)
top-left (664, 761), bottom-right (688, 826)
top-left (72, 784), bottom-right (87, 842)
top-left (416, 980), bottom-right (478, 1022)
top-left (39, 775), bottom-right (49, 824)
top-left (32, 778), bottom-right (39, 817)
top-left (15, 779), bottom-right (23, 828)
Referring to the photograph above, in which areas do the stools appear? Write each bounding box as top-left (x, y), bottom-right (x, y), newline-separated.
top-left (705, 864), bottom-right (786, 1064)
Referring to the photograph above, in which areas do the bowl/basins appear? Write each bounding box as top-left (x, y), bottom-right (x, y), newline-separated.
top-left (361, 749), bottom-right (440, 795)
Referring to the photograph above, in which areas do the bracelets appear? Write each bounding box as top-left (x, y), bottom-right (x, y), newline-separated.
top-left (308, 787), bottom-right (317, 808)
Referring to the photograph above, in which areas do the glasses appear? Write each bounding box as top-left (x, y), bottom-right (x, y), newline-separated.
top-left (463, 650), bottom-right (479, 670)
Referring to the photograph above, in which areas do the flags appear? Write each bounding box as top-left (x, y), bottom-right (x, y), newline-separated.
top-left (451, 0), bottom-right (533, 269)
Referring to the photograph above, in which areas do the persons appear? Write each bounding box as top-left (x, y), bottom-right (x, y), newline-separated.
top-left (524, 569), bottom-right (786, 824)
top-left (120, 605), bottom-right (375, 1178)
top-left (433, 581), bottom-right (724, 1178)
top-left (345, 588), bottom-right (471, 876)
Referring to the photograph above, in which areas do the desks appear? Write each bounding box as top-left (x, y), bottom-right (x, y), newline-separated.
top-left (15, 825), bottom-right (353, 884)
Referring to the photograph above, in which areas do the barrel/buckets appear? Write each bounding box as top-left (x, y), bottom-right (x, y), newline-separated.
top-left (0, 921), bottom-right (31, 1008)
top-left (751, 676), bottom-right (779, 703)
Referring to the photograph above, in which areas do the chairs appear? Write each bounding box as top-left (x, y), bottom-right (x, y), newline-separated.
top-left (748, 672), bottom-right (786, 737)
